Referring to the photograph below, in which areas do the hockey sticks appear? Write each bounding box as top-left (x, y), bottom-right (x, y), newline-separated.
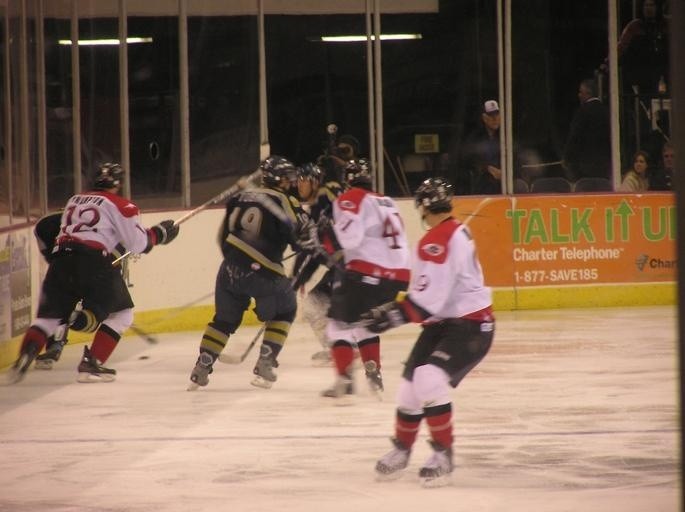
top-left (105, 170), bottom-right (262, 267)
top-left (130, 323), bottom-right (158, 346)
top-left (218, 325), bottom-right (265, 363)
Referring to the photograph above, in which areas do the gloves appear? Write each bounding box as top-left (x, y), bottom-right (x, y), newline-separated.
top-left (150, 218), bottom-right (181, 245)
top-left (298, 237), bottom-right (319, 260)
top-left (358, 301), bottom-right (410, 334)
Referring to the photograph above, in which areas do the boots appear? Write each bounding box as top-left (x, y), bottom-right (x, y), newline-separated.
top-left (9, 341), bottom-right (39, 376)
top-left (372, 436), bottom-right (412, 475)
top-left (189, 351), bottom-right (215, 387)
top-left (75, 345), bottom-right (119, 376)
top-left (416, 439), bottom-right (455, 479)
top-left (319, 361), bottom-right (355, 399)
top-left (252, 340), bottom-right (283, 383)
top-left (34, 340), bottom-right (65, 364)
top-left (362, 359), bottom-right (383, 393)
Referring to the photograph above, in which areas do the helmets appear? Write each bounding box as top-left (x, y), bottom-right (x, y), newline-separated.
top-left (296, 159), bottom-right (323, 184)
top-left (259, 154), bottom-right (297, 185)
top-left (412, 175), bottom-right (456, 212)
top-left (344, 158), bottom-right (371, 186)
top-left (93, 161), bottom-right (126, 189)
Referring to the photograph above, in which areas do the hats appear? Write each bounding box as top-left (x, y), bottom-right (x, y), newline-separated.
top-left (333, 134), bottom-right (360, 155)
top-left (483, 99), bottom-right (500, 113)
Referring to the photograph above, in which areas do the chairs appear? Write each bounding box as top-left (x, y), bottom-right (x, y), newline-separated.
top-left (398, 127), bottom-right (610, 195)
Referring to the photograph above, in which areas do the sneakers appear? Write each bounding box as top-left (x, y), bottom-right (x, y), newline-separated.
top-left (311, 350), bottom-right (335, 362)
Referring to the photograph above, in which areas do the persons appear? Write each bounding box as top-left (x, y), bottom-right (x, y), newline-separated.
top-left (321, 158), bottom-right (411, 398)
top-left (191, 156), bottom-right (309, 386)
top-left (33, 207), bottom-right (128, 360)
top-left (360, 178), bottom-right (497, 477)
top-left (15, 161), bottom-right (179, 375)
top-left (289, 163), bottom-right (344, 301)
